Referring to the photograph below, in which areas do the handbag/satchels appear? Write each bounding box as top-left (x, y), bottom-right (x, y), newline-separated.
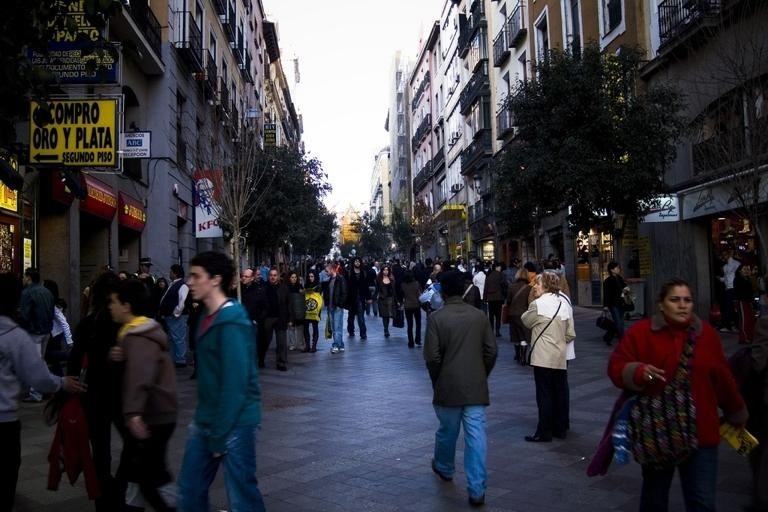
top-left (596, 316), bottom-right (614, 332)
top-left (325, 317), bottom-right (332, 339)
top-left (419, 289), bottom-right (444, 313)
top-left (393, 309), bottom-right (404, 328)
top-left (629, 380), bottom-right (697, 470)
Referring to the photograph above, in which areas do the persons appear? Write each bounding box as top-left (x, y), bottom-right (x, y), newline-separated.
top-left (578, 245), bottom-right (586, 257)
top-left (586, 278), bottom-right (748, 511)
top-left (734, 254), bottom-right (756, 344)
top-left (235, 257), bottom-right (465, 371)
top-left (2, 249), bottom-right (266, 511)
top-left (602, 261), bottom-right (634, 346)
top-left (194, 177), bottom-right (224, 238)
top-left (714, 250), bottom-right (739, 332)
top-left (459, 253), bottom-right (575, 445)
top-left (422, 267), bottom-right (497, 510)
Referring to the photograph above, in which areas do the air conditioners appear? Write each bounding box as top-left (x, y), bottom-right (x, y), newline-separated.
top-left (446, 70), bottom-right (465, 195)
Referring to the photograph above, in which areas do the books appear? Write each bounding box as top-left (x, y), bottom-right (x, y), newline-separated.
top-left (718, 422), bottom-right (759, 460)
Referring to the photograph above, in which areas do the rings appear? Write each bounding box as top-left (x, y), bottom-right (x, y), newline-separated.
top-left (648, 374), bottom-right (653, 381)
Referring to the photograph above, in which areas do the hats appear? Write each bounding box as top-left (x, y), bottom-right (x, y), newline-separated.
top-left (139, 258), bottom-right (152, 266)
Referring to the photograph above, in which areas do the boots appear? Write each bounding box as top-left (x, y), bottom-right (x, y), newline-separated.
top-left (310, 344), bottom-right (317, 353)
top-left (301, 344), bottom-right (310, 352)
top-left (514, 344), bottom-right (528, 366)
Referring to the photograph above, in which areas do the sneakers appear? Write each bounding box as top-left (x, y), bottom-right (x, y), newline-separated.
top-left (332, 346), bottom-right (344, 354)
top-left (277, 363), bottom-right (287, 370)
top-left (525, 430), bottom-right (567, 442)
top-left (289, 346), bottom-right (296, 350)
